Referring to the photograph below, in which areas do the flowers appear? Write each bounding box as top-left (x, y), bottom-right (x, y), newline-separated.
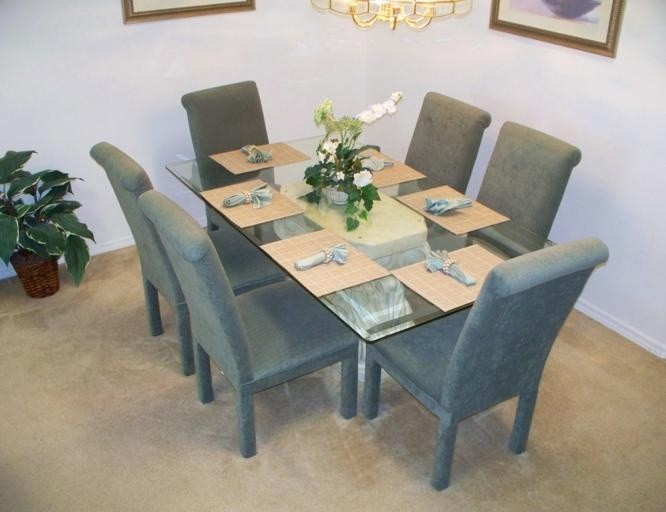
top-left (296, 88), bottom-right (401, 230)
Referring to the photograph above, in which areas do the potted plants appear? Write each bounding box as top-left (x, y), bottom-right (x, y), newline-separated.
top-left (0, 147), bottom-right (98, 299)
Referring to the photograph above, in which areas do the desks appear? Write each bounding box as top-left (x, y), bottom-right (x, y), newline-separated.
top-left (163, 130), bottom-right (561, 383)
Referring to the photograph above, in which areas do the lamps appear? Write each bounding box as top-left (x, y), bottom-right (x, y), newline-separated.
top-left (309, 0), bottom-right (474, 33)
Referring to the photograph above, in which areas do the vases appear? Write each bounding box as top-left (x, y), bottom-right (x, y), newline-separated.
top-left (320, 183), bottom-right (349, 206)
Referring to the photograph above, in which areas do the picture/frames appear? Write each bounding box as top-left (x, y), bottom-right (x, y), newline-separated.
top-left (120, 0), bottom-right (256, 26)
top-left (486, 0), bottom-right (628, 60)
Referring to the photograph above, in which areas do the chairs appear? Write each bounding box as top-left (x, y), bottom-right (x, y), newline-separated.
top-left (179, 77), bottom-right (277, 230)
top-left (474, 120), bottom-right (586, 241)
top-left (400, 90), bottom-right (493, 196)
top-left (136, 187), bottom-right (361, 460)
top-left (362, 235), bottom-right (612, 493)
top-left (88, 140), bottom-right (289, 379)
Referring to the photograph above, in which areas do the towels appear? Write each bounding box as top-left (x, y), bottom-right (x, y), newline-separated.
top-left (358, 154), bottom-right (395, 171)
top-left (239, 143), bottom-right (272, 164)
top-left (422, 245), bottom-right (477, 287)
top-left (422, 196), bottom-right (473, 215)
top-left (223, 180), bottom-right (273, 209)
top-left (292, 241), bottom-right (350, 272)
